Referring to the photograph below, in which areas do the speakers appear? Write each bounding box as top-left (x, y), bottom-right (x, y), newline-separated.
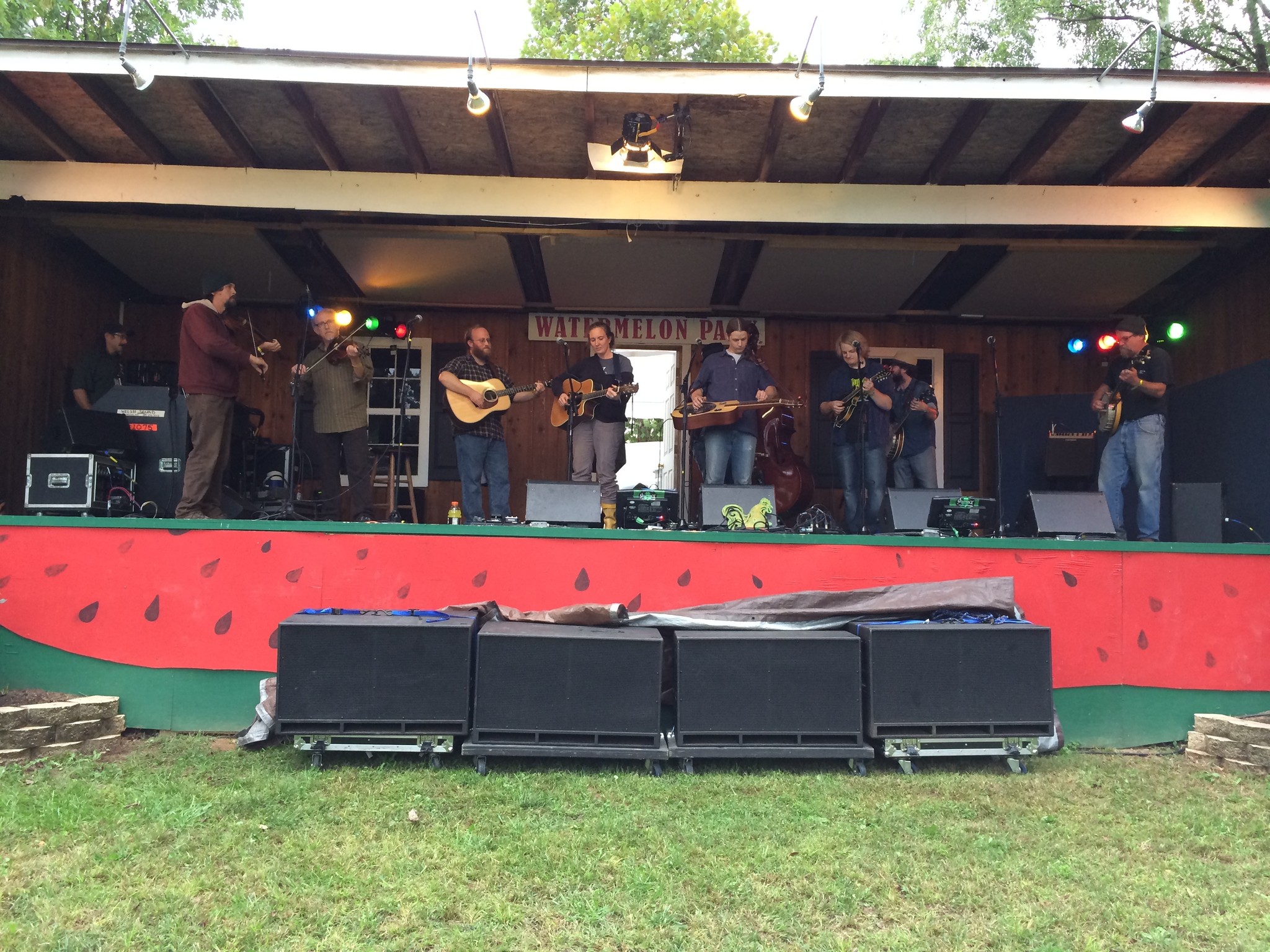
top-left (698, 483), bottom-right (778, 531)
top-left (525, 479), bottom-right (602, 528)
top-left (1018, 491), bottom-right (1116, 539)
top-left (878, 488), bottom-right (963, 536)
top-left (276, 608), bottom-right (1055, 750)
top-left (1171, 484), bottom-right (1223, 543)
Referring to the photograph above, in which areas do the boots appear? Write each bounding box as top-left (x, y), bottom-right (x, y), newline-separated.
top-left (601, 503), bottom-right (616, 529)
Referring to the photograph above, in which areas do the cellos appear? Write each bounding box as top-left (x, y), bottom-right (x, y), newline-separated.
top-left (748, 321), bottom-right (816, 523)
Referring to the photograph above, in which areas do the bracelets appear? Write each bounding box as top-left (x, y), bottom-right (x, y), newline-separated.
top-left (1134, 378), bottom-right (1142, 389)
top-left (257, 347), bottom-right (262, 353)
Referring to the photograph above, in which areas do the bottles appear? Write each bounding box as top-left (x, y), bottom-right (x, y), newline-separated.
top-left (447, 501), bottom-right (462, 525)
top-left (296, 484), bottom-right (302, 500)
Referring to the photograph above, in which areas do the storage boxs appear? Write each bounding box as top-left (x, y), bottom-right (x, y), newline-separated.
top-left (253, 446), bottom-right (315, 514)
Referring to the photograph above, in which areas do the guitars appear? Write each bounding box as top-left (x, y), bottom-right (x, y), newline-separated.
top-left (1097, 350), bottom-right (1151, 437)
top-left (671, 395), bottom-right (805, 430)
top-left (550, 378), bottom-right (639, 431)
top-left (885, 386), bottom-right (936, 462)
top-left (441, 377), bottom-right (553, 430)
top-left (832, 363), bottom-right (892, 430)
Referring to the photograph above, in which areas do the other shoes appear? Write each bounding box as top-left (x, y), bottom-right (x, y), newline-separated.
top-left (178, 512), bottom-right (213, 519)
top-left (359, 516), bottom-right (372, 522)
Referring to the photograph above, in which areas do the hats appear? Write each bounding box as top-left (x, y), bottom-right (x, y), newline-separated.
top-left (203, 268), bottom-right (234, 299)
top-left (1115, 316), bottom-right (1147, 335)
top-left (104, 321), bottom-right (134, 337)
top-left (894, 351), bottom-right (917, 365)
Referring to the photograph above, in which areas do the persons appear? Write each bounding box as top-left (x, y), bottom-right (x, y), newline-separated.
top-left (438, 325), bottom-right (546, 525)
top-left (883, 348), bottom-right (938, 488)
top-left (290, 307), bottom-right (374, 522)
top-left (1091, 314), bottom-right (1171, 542)
top-left (818, 330), bottom-right (895, 536)
top-left (551, 320), bottom-right (634, 529)
top-left (687, 317), bottom-right (777, 484)
top-left (175, 267), bottom-right (281, 520)
top-left (68, 320), bottom-right (128, 410)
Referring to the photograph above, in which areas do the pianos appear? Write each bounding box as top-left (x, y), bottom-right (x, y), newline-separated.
top-left (1045, 431), bottom-right (1094, 477)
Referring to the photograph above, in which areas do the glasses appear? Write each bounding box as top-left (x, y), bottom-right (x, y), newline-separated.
top-left (314, 319), bottom-right (335, 328)
top-left (1116, 334), bottom-right (1135, 343)
top-left (114, 333), bottom-right (129, 341)
top-left (475, 338), bottom-right (491, 344)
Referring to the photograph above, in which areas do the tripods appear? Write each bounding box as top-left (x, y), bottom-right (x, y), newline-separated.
top-left (255, 297), bottom-right (317, 521)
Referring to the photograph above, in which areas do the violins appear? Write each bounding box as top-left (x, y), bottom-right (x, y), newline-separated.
top-left (222, 310), bottom-right (283, 360)
top-left (326, 340), bottom-right (371, 364)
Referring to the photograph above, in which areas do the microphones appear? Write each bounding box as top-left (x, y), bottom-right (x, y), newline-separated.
top-left (852, 340), bottom-right (861, 350)
top-left (695, 337), bottom-right (703, 345)
top-left (407, 315), bottom-right (423, 325)
top-left (557, 337), bottom-right (571, 350)
top-left (988, 336), bottom-right (996, 344)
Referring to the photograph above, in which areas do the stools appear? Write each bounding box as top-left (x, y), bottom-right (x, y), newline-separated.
top-left (371, 447), bottom-right (418, 523)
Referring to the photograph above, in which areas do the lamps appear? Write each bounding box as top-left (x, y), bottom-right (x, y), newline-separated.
top-left (119, 0), bottom-right (190, 91)
top-left (465, 8), bottom-right (492, 115)
top-left (1097, 22), bottom-right (1161, 134)
top-left (611, 103), bottom-right (693, 167)
top-left (790, 16), bottom-right (826, 120)
top-left (379, 313), bottom-right (410, 340)
